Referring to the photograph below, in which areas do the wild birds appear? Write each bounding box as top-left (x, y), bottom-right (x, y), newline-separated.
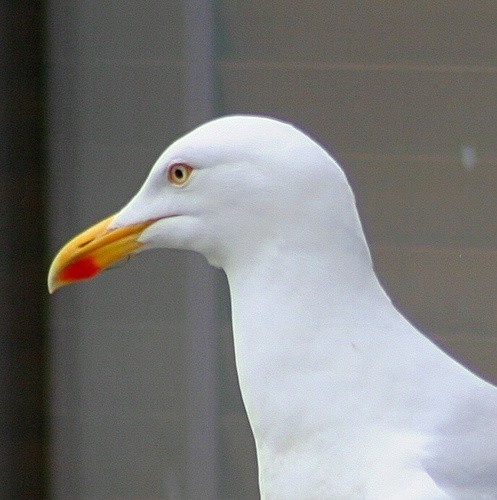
top-left (47, 115), bottom-right (496, 500)
